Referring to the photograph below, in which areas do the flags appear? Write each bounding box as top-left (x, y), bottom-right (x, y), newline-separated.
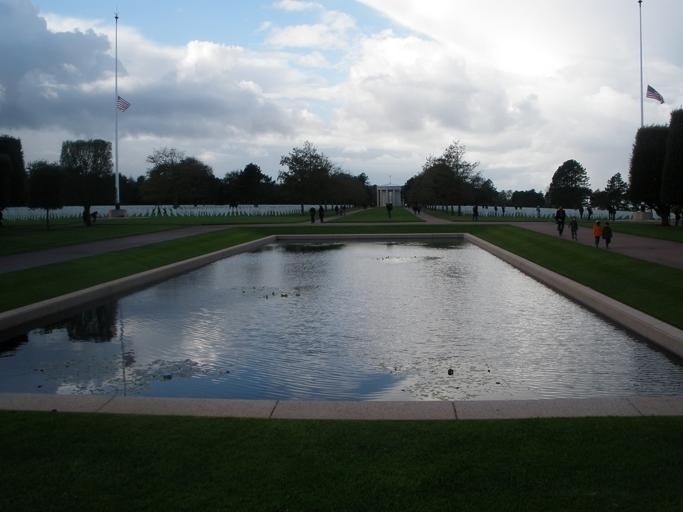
top-left (116, 96), bottom-right (129, 113)
top-left (645, 85), bottom-right (664, 105)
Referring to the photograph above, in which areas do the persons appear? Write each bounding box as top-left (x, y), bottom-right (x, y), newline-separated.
top-left (555, 205), bottom-right (565, 236)
top-left (470, 202), bottom-right (479, 222)
top-left (308, 207), bottom-right (316, 224)
top-left (601, 221), bottom-right (613, 248)
top-left (317, 206), bottom-right (324, 224)
top-left (592, 221), bottom-right (603, 248)
top-left (567, 215), bottom-right (579, 241)
